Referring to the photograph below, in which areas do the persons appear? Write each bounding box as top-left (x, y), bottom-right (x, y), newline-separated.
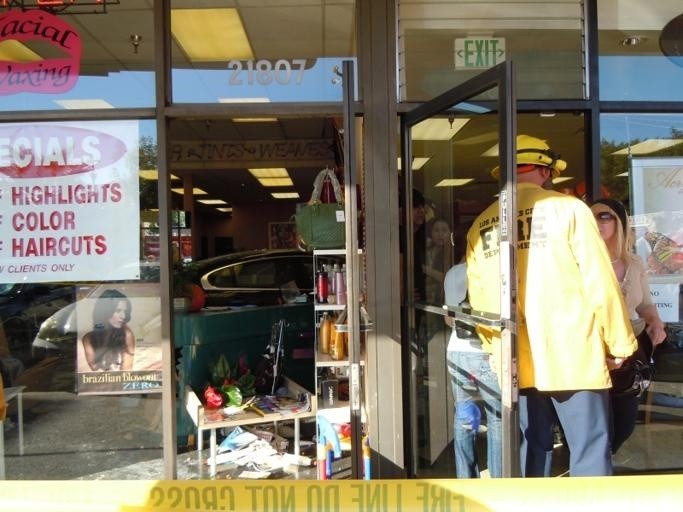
top-left (439, 241), bottom-right (529, 479)
top-left (80, 288), bottom-right (138, 372)
top-left (463, 130), bottom-right (638, 475)
top-left (394, 185), bottom-right (459, 347)
top-left (581, 196), bottom-right (667, 453)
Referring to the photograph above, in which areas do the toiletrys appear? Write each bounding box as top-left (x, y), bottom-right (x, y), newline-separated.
top-left (316, 263), bottom-right (349, 360)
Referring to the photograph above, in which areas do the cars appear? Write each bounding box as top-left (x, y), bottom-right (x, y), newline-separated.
top-left (27, 259), bottom-right (161, 375)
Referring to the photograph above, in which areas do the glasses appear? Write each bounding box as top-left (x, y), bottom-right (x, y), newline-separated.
top-left (593, 209), bottom-right (619, 227)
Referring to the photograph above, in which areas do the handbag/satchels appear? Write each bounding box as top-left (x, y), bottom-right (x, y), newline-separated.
top-left (290, 165), bottom-right (355, 252)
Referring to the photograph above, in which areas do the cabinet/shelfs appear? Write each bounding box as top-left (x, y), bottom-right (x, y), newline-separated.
top-left (312, 249), bottom-right (367, 425)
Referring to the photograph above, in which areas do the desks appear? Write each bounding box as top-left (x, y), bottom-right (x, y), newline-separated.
top-left (180, 373), bottom-right (312, 481)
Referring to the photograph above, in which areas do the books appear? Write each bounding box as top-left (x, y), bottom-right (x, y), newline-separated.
top-left (248, 391), bottom-right (312, 419)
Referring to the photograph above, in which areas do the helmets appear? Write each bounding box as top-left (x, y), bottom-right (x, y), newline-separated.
top-left (491, 133), bottom-right (567, 180)
